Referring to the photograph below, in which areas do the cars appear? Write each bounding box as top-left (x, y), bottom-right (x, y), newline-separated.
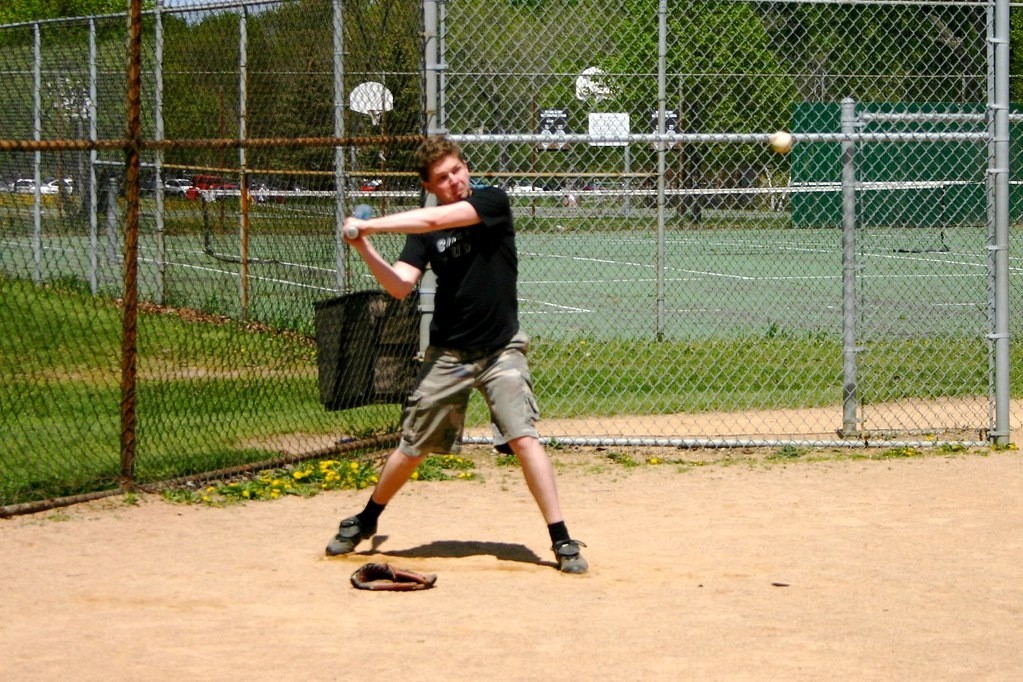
top-left (110, 173), bottom-right (287, 201)
top-left (0, 175), bottom-right (79, 196)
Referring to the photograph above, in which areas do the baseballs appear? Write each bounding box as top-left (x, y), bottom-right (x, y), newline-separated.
top-left (771, 131), bottom-right (792, 154)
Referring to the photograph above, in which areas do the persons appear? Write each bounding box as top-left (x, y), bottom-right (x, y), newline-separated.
top-left (326, 136), bottom-right (591, 574)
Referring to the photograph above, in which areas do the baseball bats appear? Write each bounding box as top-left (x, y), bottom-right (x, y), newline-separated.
top-left (346, 204), bottom-right (372, 240)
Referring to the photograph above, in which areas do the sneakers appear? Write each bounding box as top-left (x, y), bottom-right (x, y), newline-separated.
top-left (325, 514), bottom-right (377, 555)
top-left (550, 539), bottom-right (589, 574)
